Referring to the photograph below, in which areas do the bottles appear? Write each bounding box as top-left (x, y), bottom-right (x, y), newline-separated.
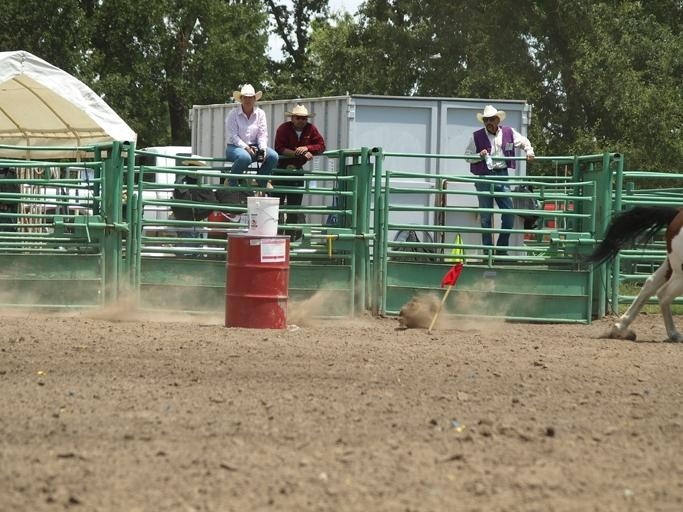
top-left (485, 153), bottom-right (493, 170)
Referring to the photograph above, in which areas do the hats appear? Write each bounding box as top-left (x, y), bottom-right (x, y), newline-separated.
top-left (233, 84), bottom-right (262, 102)
top-left (284, 104), bottom-right (316, 118)
top-left (476, 105), bottom-right (506, 123)
top-left (181, 155), bottom-right (207, 166)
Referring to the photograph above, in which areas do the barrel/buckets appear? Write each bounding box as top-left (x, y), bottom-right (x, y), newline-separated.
top-left (225, 234), bottom-right (292, 329)
top-left (247, 195), bottom-right (280, 238)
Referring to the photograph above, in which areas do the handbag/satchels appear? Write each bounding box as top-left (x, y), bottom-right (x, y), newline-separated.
top-left (170, 175), bottom-right (255, 221)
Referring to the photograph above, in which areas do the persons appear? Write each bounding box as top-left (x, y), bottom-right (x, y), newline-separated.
top-left (224, 95), bottom-right (278, 189)
top-left (169, 155), bottom-right (217, 258)
top-left (464, 117), bottom-right (535, 264)
top-left (274, 105), bottom-right (326, 238)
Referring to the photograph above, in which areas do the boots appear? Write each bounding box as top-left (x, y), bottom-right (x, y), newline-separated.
top-left (482, 244), bottom-right (517, 265)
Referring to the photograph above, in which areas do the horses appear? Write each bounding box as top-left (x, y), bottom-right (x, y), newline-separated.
top-left (582, 204), bottom-right (683, 342)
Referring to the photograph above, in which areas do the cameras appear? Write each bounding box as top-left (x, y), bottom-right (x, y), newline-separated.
top-left (252, 150), bottom-right (264, 162)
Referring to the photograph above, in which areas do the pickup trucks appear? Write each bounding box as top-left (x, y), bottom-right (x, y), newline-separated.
top-left (18, 143), bottom-right (194, 237)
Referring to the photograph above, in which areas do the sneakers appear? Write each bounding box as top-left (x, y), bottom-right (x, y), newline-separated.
top-left (256, 178), bottom-right (273, 189)
